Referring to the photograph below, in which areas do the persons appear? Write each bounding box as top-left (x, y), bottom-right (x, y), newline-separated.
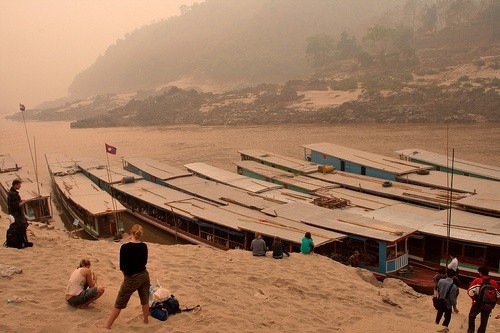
top-left (300, 231), bottom-right (315, 255)
top-left (348, 249), bottom-right (360, 267)
top-left (270, 236), bottom-right (291, 260)
top-left (250, 232), bottom-right (269, 257)
top-left (7, 179), bottom-right (34, 248)
top-left (95, 224), bottom-right (151, 330)
top-left (447, 251), bottom-right (459, 271)
top-left (466, 266), bottom-right (500, 333)
top-left (432, 268), bottom-right (461, 327)
top-left (63, 257), bottom-right (106, 310)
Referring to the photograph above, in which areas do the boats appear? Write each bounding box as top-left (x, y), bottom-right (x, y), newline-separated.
top-left (45, 153), bottom-right (125, 242)
top-left (300, 142), bottom-right (500, 201)
top-left (0, 150), bottom-right (51, 222)
top-left (233, 159), bottom-right (500, 249)
top-left (185, 161), bottom-right (500, 269)
top-left (122, 155), bottom-right (448, 289)
top-left (237, 146), bottom-right (499, 227)
top-left (393, 146), bottom-right (500, 183)
top-left (74, 153), bottom-right (347, 256)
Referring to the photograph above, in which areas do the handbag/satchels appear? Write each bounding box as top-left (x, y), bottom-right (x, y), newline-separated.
top-left (150, 301), bottom-right (168, 321)
top-left (162, 298), bottom-right (181, 315)
top-left (432, 297), bottom-right (451, 311)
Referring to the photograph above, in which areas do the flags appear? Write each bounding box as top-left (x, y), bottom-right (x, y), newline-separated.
top-left (20, 104), bottom-right (26, 111)
top-left (105, 143), bottom-right (117, 155)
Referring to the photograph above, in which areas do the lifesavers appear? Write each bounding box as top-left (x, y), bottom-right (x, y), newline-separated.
top-left (416, 169), bottom-right (429, 175)
top-left (382, 181), bottom-right (393, 187)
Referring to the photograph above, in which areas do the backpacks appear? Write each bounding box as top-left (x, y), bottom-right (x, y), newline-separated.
top-left (2, 222), bottom-right (26, 249)
top-left (474, 277), bottom-right (497, 312)
